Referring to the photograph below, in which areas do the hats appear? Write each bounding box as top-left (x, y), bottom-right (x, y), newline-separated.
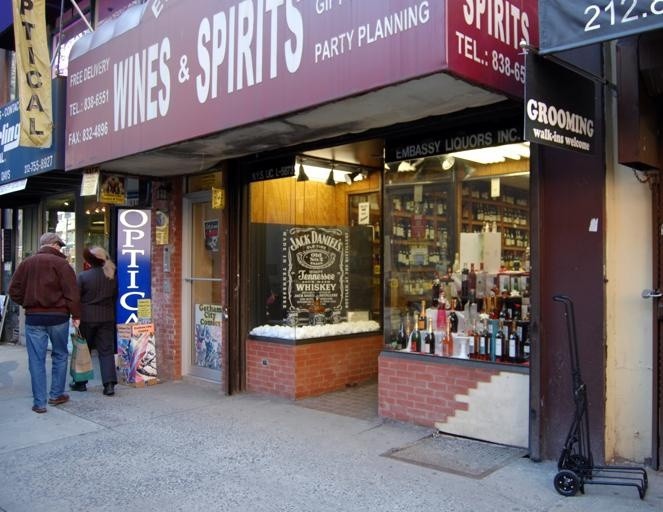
top-left (40, 233), bottom-right (66, 246)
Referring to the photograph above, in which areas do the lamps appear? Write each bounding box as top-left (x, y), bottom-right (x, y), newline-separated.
top-left (394, 154), bottom-right (458, 173)
top-left (295, 156), bottom-right (363, 188)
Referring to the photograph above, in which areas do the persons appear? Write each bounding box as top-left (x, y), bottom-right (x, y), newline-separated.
top-left (8, 232), bottom-right (118, 413)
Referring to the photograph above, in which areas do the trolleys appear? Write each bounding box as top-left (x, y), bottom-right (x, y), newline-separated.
top-left (552, 295), bottom-right (648, 500)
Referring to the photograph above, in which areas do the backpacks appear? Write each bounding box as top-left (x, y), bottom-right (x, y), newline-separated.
top-left (69, 333), bottom-right (93, 381)
top-left (103, 386), bottom-right (114, 395)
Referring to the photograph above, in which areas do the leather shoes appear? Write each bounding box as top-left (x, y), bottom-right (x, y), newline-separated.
top-left (32, 407), bottom-right (46, 413)
top-left (47, 394), bottom-right (68, 404)
top-left (69, 384), bottom-right (87, 391)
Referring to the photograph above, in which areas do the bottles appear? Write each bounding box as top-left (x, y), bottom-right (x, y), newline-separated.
top-left (388, 195), bottom-right (532, 362)
top-left (310, 290), bottom-right (325, 326)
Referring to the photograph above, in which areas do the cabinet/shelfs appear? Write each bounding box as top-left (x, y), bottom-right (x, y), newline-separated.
top-left (349, 180), bottom-right (531, 318)
